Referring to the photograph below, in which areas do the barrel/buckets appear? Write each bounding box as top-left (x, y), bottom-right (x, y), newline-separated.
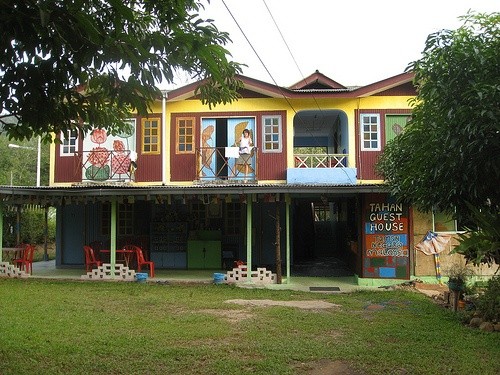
top-left (135, 273), bottom-right (148, 282)
top-left (214, 272), bottom-right (225, 285)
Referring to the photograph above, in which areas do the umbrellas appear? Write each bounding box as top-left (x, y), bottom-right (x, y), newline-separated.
top-left (414, 230), bottom-right (454, 283)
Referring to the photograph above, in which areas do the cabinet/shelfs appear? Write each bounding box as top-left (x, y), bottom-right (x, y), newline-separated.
top-left (150, 204), bottom-right (187, 269)
top-left (188, 241), bottom-right (221, 269)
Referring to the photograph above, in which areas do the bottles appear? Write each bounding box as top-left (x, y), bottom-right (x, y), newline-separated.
top-left (153, 234), bottom-right (185, 252)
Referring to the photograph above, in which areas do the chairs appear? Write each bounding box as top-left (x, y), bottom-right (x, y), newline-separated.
top-left (136, 246), bottom-right (154, 277)
top-left (83, 246), bottom-right (103, 273)
top-left (13, 242), bottom-right (34, 275)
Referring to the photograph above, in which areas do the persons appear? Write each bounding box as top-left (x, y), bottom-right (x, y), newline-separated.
top-left (230, 129), bottom-right (253, 184)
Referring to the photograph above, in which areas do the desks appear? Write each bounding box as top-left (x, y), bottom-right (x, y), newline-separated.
top-left (2, 248), bottom-right (25, 264)
top-left (99, 250), bottom-right (135, 267)
